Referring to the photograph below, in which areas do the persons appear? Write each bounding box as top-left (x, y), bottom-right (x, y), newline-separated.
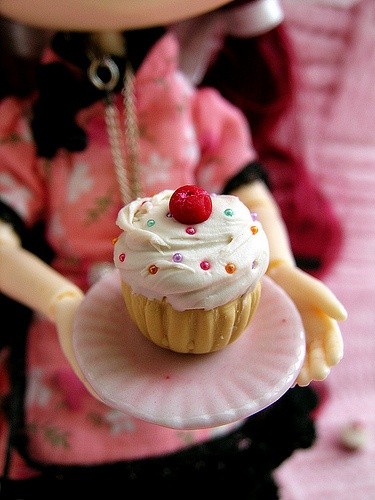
top-left (0, 1), bottom-right (348, 500)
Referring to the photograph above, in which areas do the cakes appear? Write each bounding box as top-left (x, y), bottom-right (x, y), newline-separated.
top-left (113, 184), bottom-right (270, 354)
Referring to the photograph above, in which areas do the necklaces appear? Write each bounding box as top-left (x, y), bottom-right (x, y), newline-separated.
top-left (88, 49), bottom-right (138, 214)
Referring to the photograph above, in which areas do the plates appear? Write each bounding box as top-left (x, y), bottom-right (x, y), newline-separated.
top-left (72, 266), bottom-right (308, 431)
